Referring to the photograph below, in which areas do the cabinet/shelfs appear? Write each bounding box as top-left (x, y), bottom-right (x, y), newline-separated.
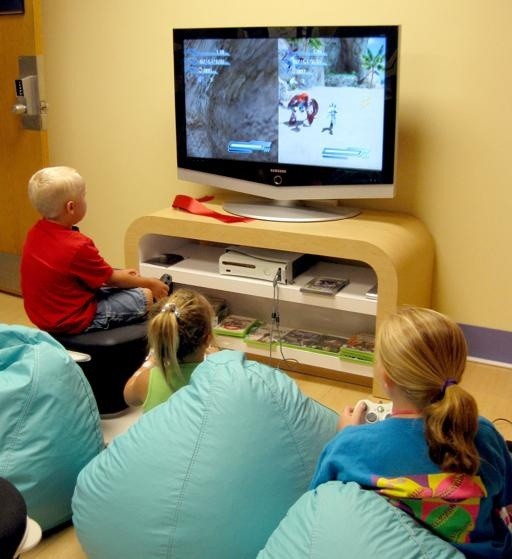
top-left (124, 199), bottom-right (436, 399)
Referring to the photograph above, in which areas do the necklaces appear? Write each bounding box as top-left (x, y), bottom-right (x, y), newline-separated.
top-left (388, 408), bottom-right (423, 416)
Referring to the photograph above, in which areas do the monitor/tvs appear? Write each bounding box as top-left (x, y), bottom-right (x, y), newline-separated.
top-left (173, 25), bottom-right (399, 223)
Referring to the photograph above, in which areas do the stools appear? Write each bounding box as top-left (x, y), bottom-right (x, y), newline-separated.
top-left (49, 317), bottom-right (149, 417)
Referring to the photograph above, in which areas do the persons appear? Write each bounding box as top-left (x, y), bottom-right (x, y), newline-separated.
top-left (306, 305), bottom-right (512, 559)
top-left (20, 165), bottom-right (173, 335)
top-left (123, 286), bottom-right (221, 414)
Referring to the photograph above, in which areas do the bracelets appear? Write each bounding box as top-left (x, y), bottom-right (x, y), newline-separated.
top-left (142, 361), bottom-right (155, 368)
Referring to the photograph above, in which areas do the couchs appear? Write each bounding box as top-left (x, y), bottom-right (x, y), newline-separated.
top-left (0, 323), bottom-right (104, 534)
top-left (70, 350), bottom-right (340, 558)
top-left (255, 480), bottom-right (467, 558)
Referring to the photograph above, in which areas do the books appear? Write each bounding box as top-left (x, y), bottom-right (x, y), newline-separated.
top-left (211, 307), bottom-right (229, 326)
top-left (301, 275), bottom-right (349, 295)
top-left (204, 295), bottom-right (226, 316)
top-left (338, 352), bottom-right (375, 366)
top-left (244, 323), bottom-right (294, 349)
top-left (306, 335), bottom-right (349, 357)
top-left (339, 332), bottom-right (378, 361)
top-left (366, 282), bottom-right (378, 298)
top-left (278, 330), bottom-right (320, 351)
top-left (213, 314), bottom-right (259, 337)
top-left (341, 332), bottom-right (382, 357)
